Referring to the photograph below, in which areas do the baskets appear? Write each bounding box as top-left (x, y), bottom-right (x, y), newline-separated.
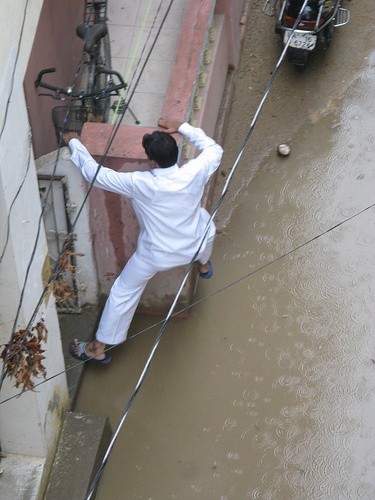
top-left (52, 106), bottom-right (105, 146)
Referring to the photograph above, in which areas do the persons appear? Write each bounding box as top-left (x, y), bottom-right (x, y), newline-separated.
top-left (62, 115), bottom-right (225, 364)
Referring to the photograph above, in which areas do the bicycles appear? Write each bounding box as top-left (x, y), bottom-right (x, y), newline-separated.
top-left (33, 0), bottom-right (141, 147)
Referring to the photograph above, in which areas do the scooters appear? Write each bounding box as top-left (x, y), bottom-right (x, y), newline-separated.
top-left (262, 0), bottom-right (352, 75)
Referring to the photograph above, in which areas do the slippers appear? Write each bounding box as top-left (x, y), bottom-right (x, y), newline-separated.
top-left (200, 260), bottom-right (212, 279)
top-left (70, 342), bottom-right (112, 365)
